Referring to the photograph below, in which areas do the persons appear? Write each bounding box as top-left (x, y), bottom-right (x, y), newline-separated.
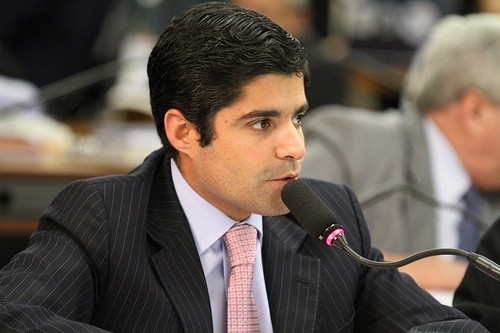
top-left (0, 2), bottom-right (486, 333)
top-left (297, 10), bottom-right (499, 290)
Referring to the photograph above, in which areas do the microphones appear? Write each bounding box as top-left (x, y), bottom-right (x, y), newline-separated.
top-left (280, 179), bottom-right (500, 281)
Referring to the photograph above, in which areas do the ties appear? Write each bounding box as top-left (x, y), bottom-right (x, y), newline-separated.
top-left (220, 224), bottom-right (263, 333)
